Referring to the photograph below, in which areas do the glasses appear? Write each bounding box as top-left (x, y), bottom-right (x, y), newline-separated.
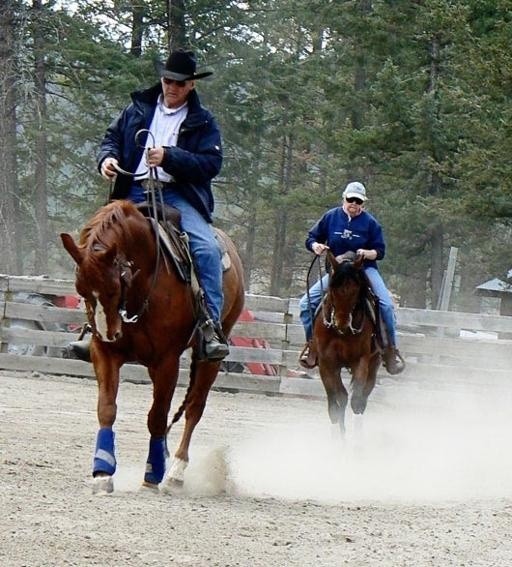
top-left (162, 77), bottom-right (188, 87)
top-left (344, 197), bottom-right (364, 204)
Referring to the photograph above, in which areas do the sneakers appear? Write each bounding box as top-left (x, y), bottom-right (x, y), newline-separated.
top-left (67, 338), bottom-right (93, 363)
top-left (383, 346), bottom-right (402, 374)
top-left (202, 336), bottom-right (230, 358)
top-left (299, 352), bottom-right (318, 369)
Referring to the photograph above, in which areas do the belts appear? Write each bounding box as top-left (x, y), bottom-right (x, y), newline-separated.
top-left (131, 178), bottom-right (177, 192)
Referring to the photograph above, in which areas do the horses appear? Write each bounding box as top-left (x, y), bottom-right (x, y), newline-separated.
top-left (59, 198), bottom-right (246, 498)
top-left (311, 248), bottom-right (384, 439)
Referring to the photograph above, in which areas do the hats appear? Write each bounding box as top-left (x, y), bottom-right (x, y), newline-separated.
top-left (152, 47), bottom-right (215, 80)
top-left (342, 181), bottom-right (368, 201)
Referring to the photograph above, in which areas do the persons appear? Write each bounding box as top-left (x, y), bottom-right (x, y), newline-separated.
top-left (70, 49), bottom-right (229, 363)
top-left (300, 182), bottom-right (403, 375)
